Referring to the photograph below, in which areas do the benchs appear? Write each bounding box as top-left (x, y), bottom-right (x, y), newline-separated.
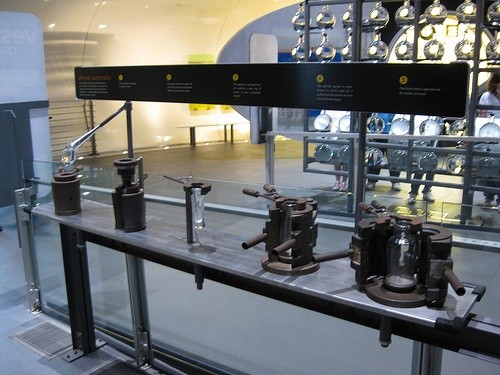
top-left (177, 123), bottom-right (240, 147)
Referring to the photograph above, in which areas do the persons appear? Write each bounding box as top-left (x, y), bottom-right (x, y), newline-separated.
top-left (477, 73), bottom-right (500, 211)
top-left (406, 117), bottom-right (445, 204)
top-left (330, 111), bottom-right (350, 192)
top-left (365, 112), bottom-right (402, 192)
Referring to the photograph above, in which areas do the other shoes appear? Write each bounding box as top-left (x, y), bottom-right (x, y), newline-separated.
top-left (484, 197), bottom-right (492, 206)
top-left (393, 183), bottom-right (402, 191)
top-left (367, 182), bottom-right (377, 189)
top-left (423, 192), bottom-right (435, 202)
top-left (339, 182), bottom-right (347, 192)
top-left (333, 181), bottom-right (340, 191)
top-left (408, 194), bottom-right (417, 204)
